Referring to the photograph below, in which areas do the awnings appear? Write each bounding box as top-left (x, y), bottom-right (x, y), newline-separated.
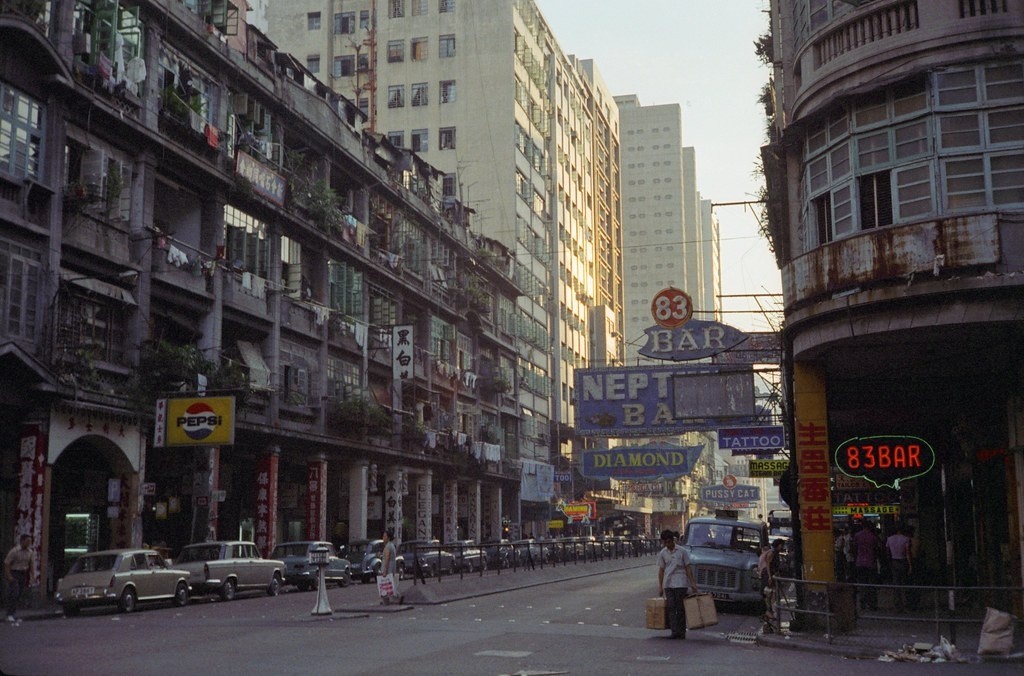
top-left (500, 411), bottom-right (527, 422)
top-left (58, 266), bottom-right (137, 305)
top-left (149, 299), bottom-right (199, 335)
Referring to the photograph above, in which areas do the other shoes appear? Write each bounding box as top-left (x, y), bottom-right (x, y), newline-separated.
top-left (870, 607), bottom-right (878, 614)
top-left (14, 615), bottom-right (22, 622)
top-left (903, 610), bottom-right (919, 615)
top-left (858, 608), bottom-right (865, 615)
top-left (865, 608), bottom-right (870, 613)
top-left (887, 607), bottom-right (894, 615)
top-left (879, 607), bottom-right (887, 616)
top-left (7, 615), bottom-right (15, 622)
top-left (895, 611), bottom-right (906, 615)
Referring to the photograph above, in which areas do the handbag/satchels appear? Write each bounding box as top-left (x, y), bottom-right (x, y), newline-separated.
top-left (376, 574), bottom-right (394, 599)
top-left (394, 573), bottom-right (401, 598)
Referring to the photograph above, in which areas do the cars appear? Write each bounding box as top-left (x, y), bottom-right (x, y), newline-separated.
top-left (56, 549), bottom-right (192, 615)
top-left (396, 540), bottom-right (458, 576)
top-left (339, 541), bottom-right (405, 584)
top-left (176, 540), bottom-right (285, 598)
top-left (544, 536), bottom-right (655, 562)
top-left (446, 541), bottom-right (489, 570)
top-left (480, 539), bottom-right (524, 568)
top-left (766, 536), bottom-right (796, 571)
top-left (272, 542), bottom-right (354, 591)
top-left (680, 518), bottom-right (773, 606)
top-left (512, 540), bottom-right (549, 564)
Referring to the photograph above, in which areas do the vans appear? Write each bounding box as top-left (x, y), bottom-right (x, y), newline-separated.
top-left (769, 509), bottom-right (796, 539)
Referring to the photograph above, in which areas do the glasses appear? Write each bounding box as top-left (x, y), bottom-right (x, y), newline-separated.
top-left (143, 545), bottom-right (147, 548)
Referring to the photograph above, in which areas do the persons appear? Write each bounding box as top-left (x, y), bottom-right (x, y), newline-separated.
top-left (656, 529), bottom-right (698, 640)
top-left (509, 527), bottom-right (653, 556)
top-left (831, 523), bottom-right (925, 612)
top-left (3, 533), bottom-right (36, 623)
top-left (378, 530), bottom-right (404, 606)
top-left (757, 546), bottom-right (771, 578)
top-left (761, 537), bottom-right (784, 621)
top-left (141, 541), bottom-right (157, 568)
